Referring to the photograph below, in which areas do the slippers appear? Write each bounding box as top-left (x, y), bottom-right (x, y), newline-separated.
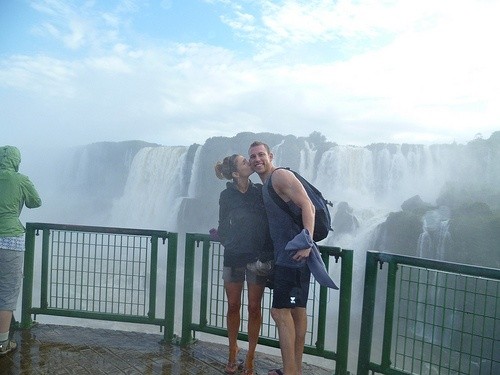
top-left (268, 366), bottom-right (284, 375)
top-left (224, 351), bottom-right (244, 375)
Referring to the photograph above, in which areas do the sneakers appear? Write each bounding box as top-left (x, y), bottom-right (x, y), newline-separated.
top-left (0, 340), bottom-right (18, 356)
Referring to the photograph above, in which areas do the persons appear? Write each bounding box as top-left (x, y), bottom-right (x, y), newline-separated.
top-left (0, 146), bottom-right (42, 354)
top-left (218, 153), bottom-right (275, 375)
top-left (249, 142), bottom-right (315, 375)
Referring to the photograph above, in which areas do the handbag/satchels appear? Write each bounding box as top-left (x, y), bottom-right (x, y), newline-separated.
top-left (256, 254), bottom-right (273, 273)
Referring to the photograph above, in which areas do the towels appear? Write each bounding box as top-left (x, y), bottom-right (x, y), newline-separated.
top-left (285, 229), bottom-right (339, 290)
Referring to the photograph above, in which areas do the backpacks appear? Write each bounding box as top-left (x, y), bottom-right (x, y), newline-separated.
top-left (267, 166), bottom-right (332, 243)
